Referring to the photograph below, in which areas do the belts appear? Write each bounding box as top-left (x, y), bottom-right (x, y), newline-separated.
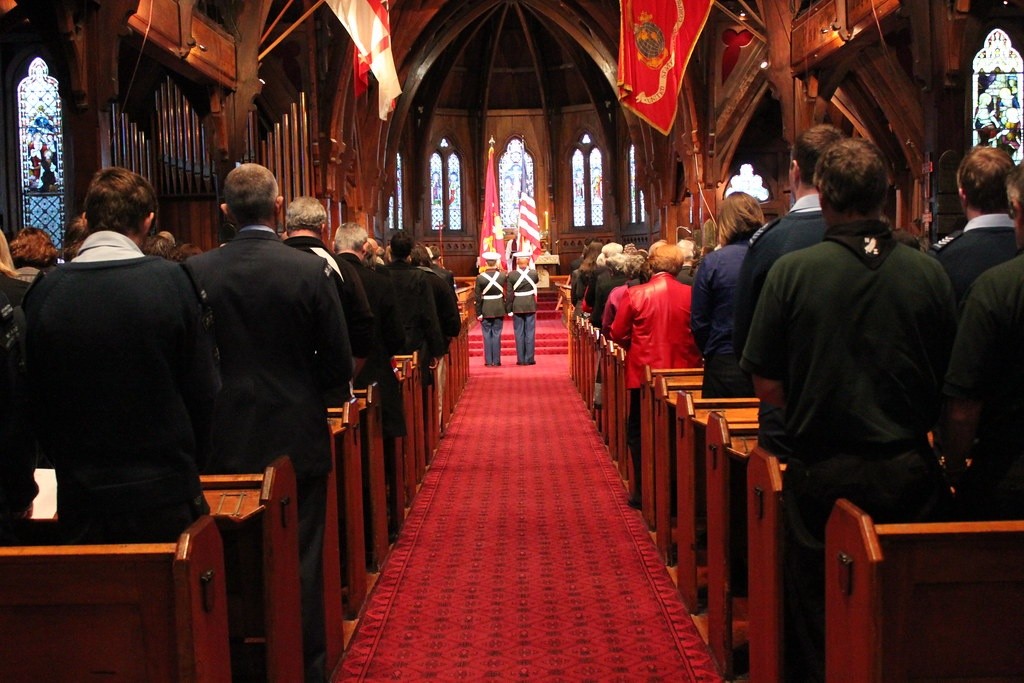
top-left (514, 290), bottom-right (534, 296)
top-left (483, 294), bottom-right (502, 300)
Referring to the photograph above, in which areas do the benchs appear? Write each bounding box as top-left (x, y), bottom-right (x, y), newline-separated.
top-left (566, 262), bottom-right (1024, 683)
top-left (0, 279), bottom-right (488, 683)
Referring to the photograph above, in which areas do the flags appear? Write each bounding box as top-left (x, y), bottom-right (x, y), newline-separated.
top-left (479, 144), bottom-right (508, 274)
top-left (616, 0), bottom-right (716, 136)
top-left (327, 0), bottom-right (402, 120)
top-left (516, 145), bottom-right (541, 271)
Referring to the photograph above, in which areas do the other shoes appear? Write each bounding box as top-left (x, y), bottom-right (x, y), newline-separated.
top-left (516, 361), bottom-right (536, 365)
top-left (627, 497), bottom-right (642, 510)
top-left (484, 362), bottom-right (501, 367)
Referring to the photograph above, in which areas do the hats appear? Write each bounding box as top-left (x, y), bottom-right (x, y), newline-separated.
top-left (513, 252), bottom-right (531, 258)
top-left (482, 252), bottom-right (501, 260)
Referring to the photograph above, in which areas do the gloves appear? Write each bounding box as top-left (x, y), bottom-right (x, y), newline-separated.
top-left (508, 312), bottom-right (513, 316)
top-left (477, 314), bottom-right (483, 320)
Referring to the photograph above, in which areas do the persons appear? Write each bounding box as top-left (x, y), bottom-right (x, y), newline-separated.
top-left (731, 122), bottom-right (1024, 683)
top-left (474, 251), bottom-right (539, 366)
top-left (0, 168), bottom-right (225, 544)
top-left (568, 190), bottom-right (766, 509)
top-left (181, 163), bottom-right (429, 683)
top-left (334, 222), bottom-right (461, 545)
